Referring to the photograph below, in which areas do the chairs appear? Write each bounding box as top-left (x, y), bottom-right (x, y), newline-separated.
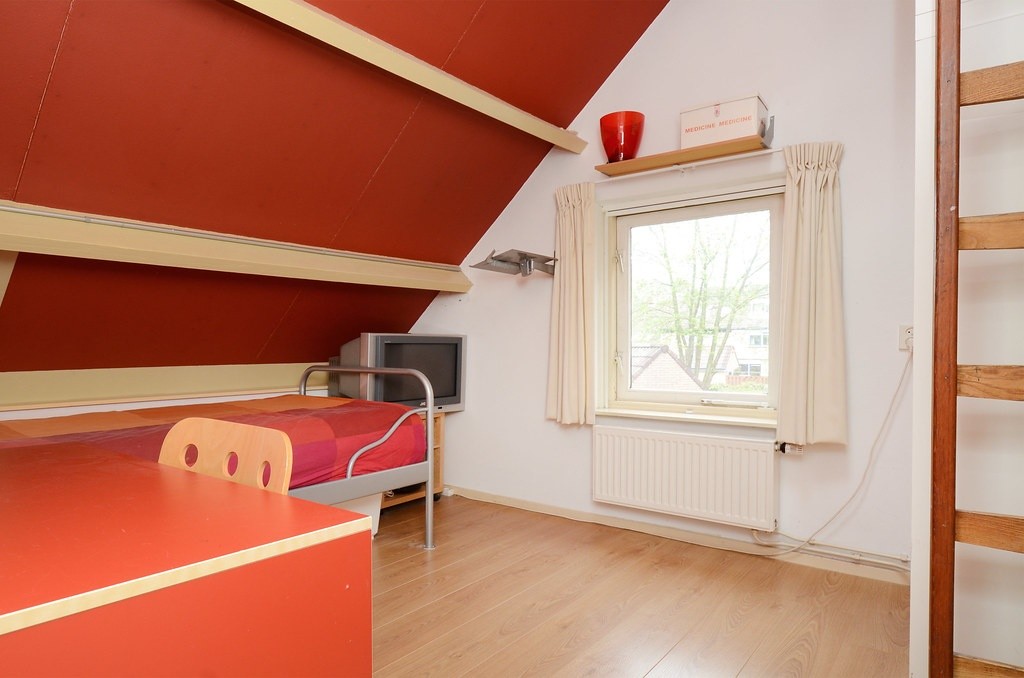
top-left (158, 417), bottom-right (293, 495)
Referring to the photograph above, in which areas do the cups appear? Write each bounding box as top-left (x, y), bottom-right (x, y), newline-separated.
top-left (599, 111), bottom-right (645, 166)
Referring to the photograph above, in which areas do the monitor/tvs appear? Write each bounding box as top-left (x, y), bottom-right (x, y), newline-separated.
top-left (338, 333), bottom-right (467, 415)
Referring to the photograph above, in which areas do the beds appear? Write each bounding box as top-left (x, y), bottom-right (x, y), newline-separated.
top-left (1, 365), bottom-right (445, 550)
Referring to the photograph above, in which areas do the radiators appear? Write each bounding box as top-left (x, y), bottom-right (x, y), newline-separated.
top-left (592, 426), bottom-right (782, 535)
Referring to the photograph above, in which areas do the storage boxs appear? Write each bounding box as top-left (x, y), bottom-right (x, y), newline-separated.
top-left (678, 95), bottom-right (765, 148)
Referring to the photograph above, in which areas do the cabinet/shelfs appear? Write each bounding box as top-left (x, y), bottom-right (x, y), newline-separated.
top-left (381, 412), bottom-right (446, 510)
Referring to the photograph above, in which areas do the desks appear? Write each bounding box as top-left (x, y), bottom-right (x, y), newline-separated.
top-left (0, 441), bottom-right (373, 676)
